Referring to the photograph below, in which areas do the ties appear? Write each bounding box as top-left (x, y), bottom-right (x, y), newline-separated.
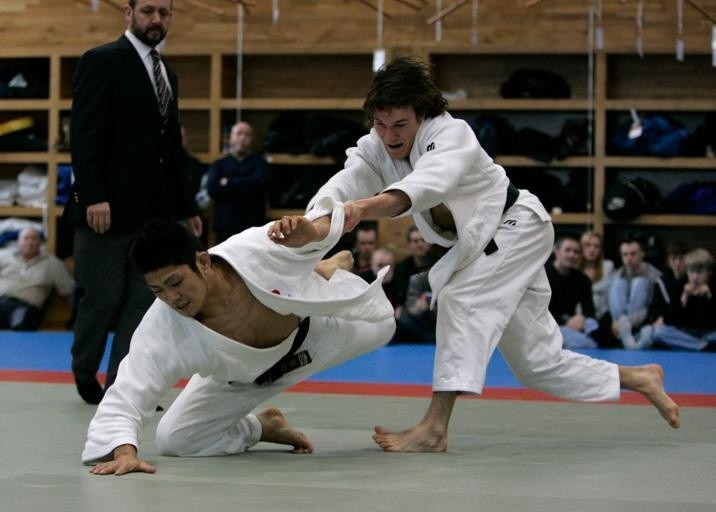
top-left (149, 48), bottom-right (171, 116)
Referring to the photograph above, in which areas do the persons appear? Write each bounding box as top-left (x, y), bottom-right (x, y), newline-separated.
top-left (305, 55), bottom-right (681, 453)
top-left (68, 1), bottom-right (203, 412)
top-left (198, 121), bottom-right (273, 242)
top-left (178, 122), bottom-right (202, 199)
top-left (342, 222), bottom-right (443, 347)
top-left (79, 195), bottom-right (398, 477)
top-left (544, 229), bottom-right (716, 351)
top-left (0, 229), bottom-right (74, 332)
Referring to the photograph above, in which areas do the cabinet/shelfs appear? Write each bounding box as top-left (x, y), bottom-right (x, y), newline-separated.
top-left (0, 50), bottom-right (716, 258)
